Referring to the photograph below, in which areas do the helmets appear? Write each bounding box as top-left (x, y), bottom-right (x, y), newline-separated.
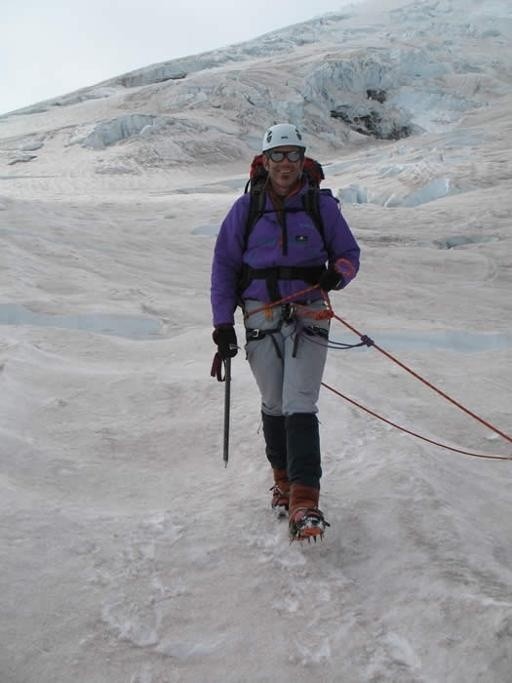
top-left (262, 124), bottom-right (306, 154)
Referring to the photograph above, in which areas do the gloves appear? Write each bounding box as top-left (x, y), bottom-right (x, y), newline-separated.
top-left (311, 268), bottom-right (342, 292)
top-left (212, 325), bottom-right (237, 357)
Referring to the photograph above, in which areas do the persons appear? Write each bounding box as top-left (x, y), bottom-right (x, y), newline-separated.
top-left (210, 122), bottom-right (362, 540)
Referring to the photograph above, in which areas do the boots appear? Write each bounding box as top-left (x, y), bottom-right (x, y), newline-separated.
top-left (291, 481), bottom-right (326, 542)
top-left (271, 466), bottom-right (291, 516)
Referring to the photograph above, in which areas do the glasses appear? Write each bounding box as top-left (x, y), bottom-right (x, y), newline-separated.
top-left (268, 150), bottom-right (303, 162)
